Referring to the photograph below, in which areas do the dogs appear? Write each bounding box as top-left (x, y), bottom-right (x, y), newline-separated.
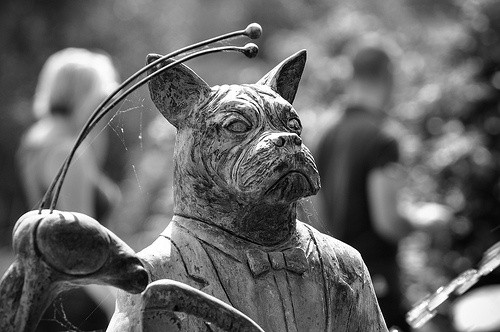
top-left (146, 48), bottom-right (323, 245)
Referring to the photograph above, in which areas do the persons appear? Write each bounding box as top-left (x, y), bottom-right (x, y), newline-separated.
top-left (312, 42), bottom-right (452, 332)
top-left (17, 46), bottom-right (124, 233)
top-left (117, 111), bottom-right (180, 259)
